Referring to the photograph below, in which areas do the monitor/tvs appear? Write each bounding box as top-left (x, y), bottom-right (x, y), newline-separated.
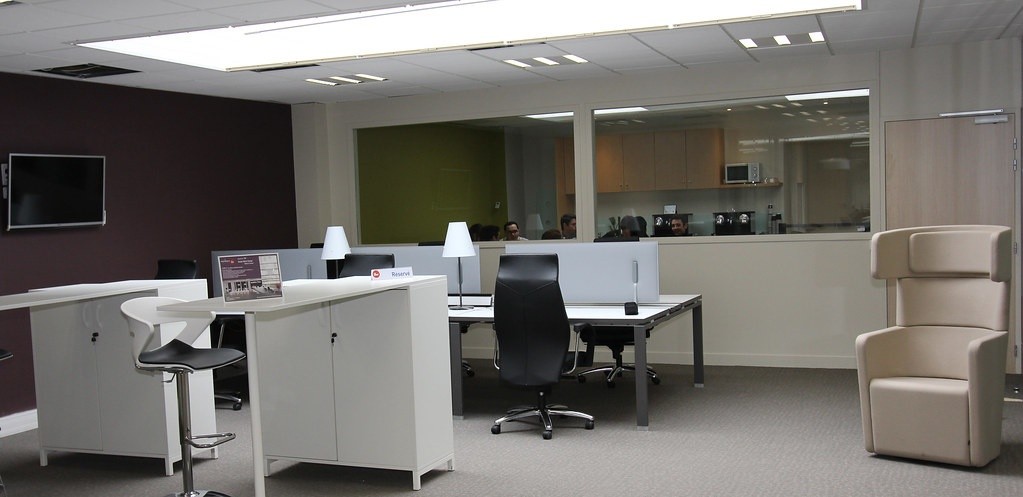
top-left (7, 153), bottom-right (106, 229)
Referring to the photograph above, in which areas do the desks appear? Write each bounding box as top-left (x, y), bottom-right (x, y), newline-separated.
top-left (0, 281), bottom-right (160, 312)
top-left (446, 295), bottom-right (706, 429)
top-left (156, 273), bottom-right (448, 313)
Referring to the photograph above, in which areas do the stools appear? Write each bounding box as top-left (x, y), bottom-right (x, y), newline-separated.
top-left (119, 296), bottom-right (246, 497)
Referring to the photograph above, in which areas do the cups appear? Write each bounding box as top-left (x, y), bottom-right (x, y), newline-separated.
top-left (767, 204), bottom-right (784, 234)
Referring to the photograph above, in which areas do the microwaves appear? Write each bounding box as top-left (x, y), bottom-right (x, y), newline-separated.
top-left (725, 162), bottom-right (760, 183)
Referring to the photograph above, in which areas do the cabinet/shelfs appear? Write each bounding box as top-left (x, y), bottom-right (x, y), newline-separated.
top-left (257, 274), bottom-right (456, 491)
top-left (28, 279), bottom-right (221, 477)
top-left (565, 128), bottom-right (724, 195)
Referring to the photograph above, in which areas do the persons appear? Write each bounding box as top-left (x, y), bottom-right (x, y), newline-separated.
top-left (672, 215), bottom-right (688, 236)
top-left (469, 214), bottom-right (649, 241)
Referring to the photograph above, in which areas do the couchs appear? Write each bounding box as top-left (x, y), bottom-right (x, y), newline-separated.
top-left (854, 224), bottom-right (1013, 467)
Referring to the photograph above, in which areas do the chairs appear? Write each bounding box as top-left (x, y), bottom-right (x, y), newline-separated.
top-left (418, 241), bottom-right (476, 379)
top-left (155, 257), bottom-right (197, 283)
top-left (578, 236), bottom-right (660, 390)
top-left (491, 253), bottom-right (595, 440)
top-left (337, 254), bottom-right (396, 278)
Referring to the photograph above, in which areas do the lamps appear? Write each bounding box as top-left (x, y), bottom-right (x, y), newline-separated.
top-left (321, 225), bottom-right (351, 279)
top-left (442, 222), bottom-right (476, 310)
